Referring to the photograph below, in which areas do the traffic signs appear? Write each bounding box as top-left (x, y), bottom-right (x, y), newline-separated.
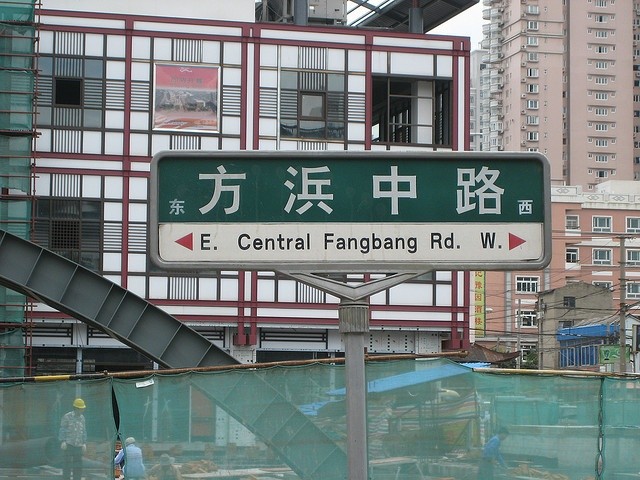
top-left (149, 151), bottom-right (552, 270)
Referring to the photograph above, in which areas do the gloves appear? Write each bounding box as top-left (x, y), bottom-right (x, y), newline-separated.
top-left (81, 443), bottom-right (86, 455)
top-left (61, 441), bottom-right (68, 451)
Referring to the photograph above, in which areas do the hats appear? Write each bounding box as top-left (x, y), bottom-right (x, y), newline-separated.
top-left (160, 454), bottom-right (175, 465)
top-left (126, 437), bottom-right (135, 446)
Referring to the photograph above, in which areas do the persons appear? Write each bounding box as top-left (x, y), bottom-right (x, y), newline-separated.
top-left (112, 437), bottom-right (146, 479)
top-left (145, 454), bottom-right (183, 480)
top-left (58, 398), bottom-right (88, 480)
top-left (477, 427), bottom-right (510, 480)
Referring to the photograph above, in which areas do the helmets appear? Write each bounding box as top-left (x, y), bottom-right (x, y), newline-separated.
top-left (72, 398), bottom-right (86, 409)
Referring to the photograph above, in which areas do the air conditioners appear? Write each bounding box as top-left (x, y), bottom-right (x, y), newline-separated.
top-left (521, 44), bottom-right (526, 49)
top-left (521, 124), bottom-right (527, 130)
top-left (521, 61), bottom-right (526, 67)
top-left (521, 0), bottom-right (527, 4)
top-left (521, 110), bottom-right (526, 114)
top-left (521, 13), bottom-right (526, 18)
top-left (521, 94), bottom-right (526, 98)
top-left (521, 27), bottom-right (526, 33)
top-left (521, 78), bottom-right (526, 82)
top-left (521, 140), bottom-right (526, 146)
